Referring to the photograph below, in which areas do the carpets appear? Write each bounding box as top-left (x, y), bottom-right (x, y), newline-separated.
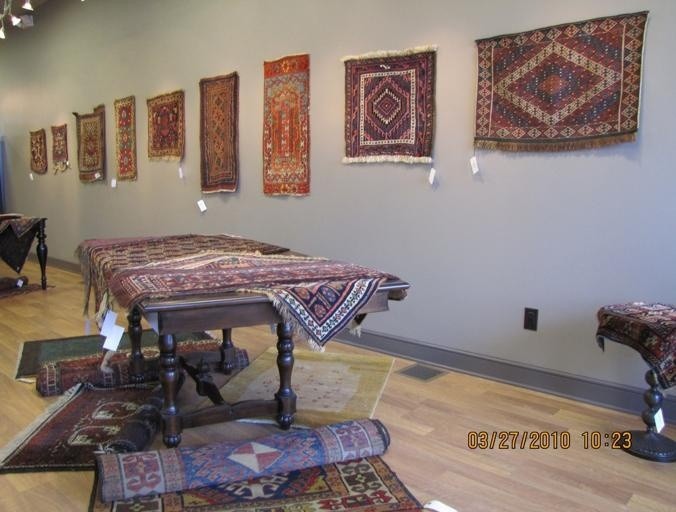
top-left (87, 418), bottom-right (424, 512)
top-left (15, 328), bottom-right (251, 397)
top-left (217, 345), bottom-right (397, 420)
top-left (0, 373), bottom-right (187, 473)
top-left (0, 276), bottom-right (56, 299)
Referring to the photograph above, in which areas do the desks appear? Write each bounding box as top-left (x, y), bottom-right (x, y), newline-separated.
top-left (0, 218), bottom-right (48, 289)
top-left (596, 300), bottom-right (676, 461)
top-left (74, 234), bottom-right (411, 451)
